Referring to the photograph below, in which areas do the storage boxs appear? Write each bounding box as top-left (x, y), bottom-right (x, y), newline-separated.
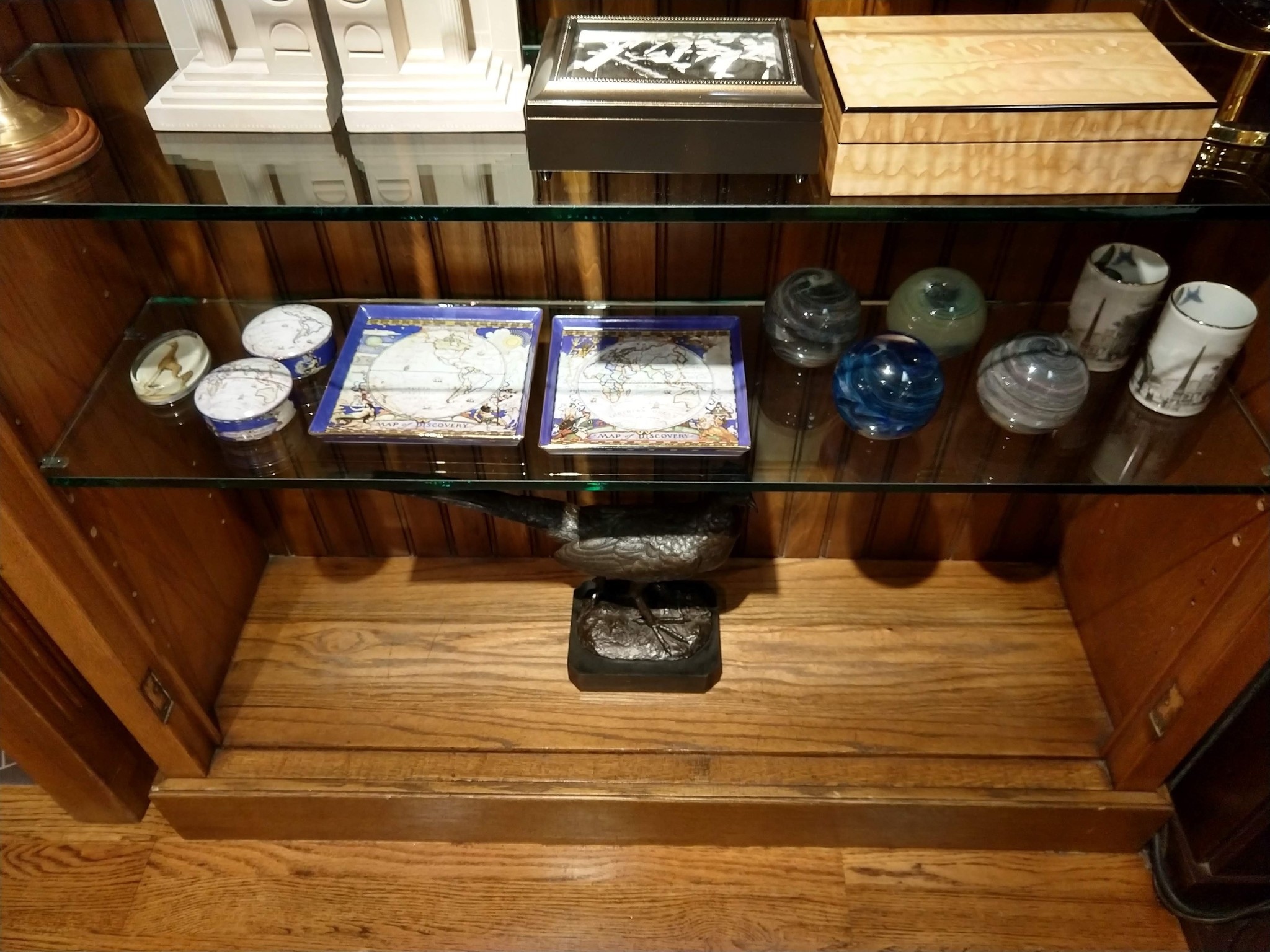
top-left (809, 12), bottom-right (1218, 196)
top-left (524, 13), bottom-right (824, 185)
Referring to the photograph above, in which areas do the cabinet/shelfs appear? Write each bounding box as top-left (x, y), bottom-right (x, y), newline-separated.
top-left (0, 42), bottom-right (1270, 845)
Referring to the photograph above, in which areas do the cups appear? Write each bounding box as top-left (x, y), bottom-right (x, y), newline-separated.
top-left (1060, 242), bottom-right (1170, 373)
top-left (1129, 279), bottom-right (1258, 418)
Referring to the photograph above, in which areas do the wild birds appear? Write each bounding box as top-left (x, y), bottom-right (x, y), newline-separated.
top-left (319, 457), bottom-right (756, 659)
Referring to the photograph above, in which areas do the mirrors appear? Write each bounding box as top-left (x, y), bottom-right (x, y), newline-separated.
top-left (1166, 0), bottom-right (1270, 148)
